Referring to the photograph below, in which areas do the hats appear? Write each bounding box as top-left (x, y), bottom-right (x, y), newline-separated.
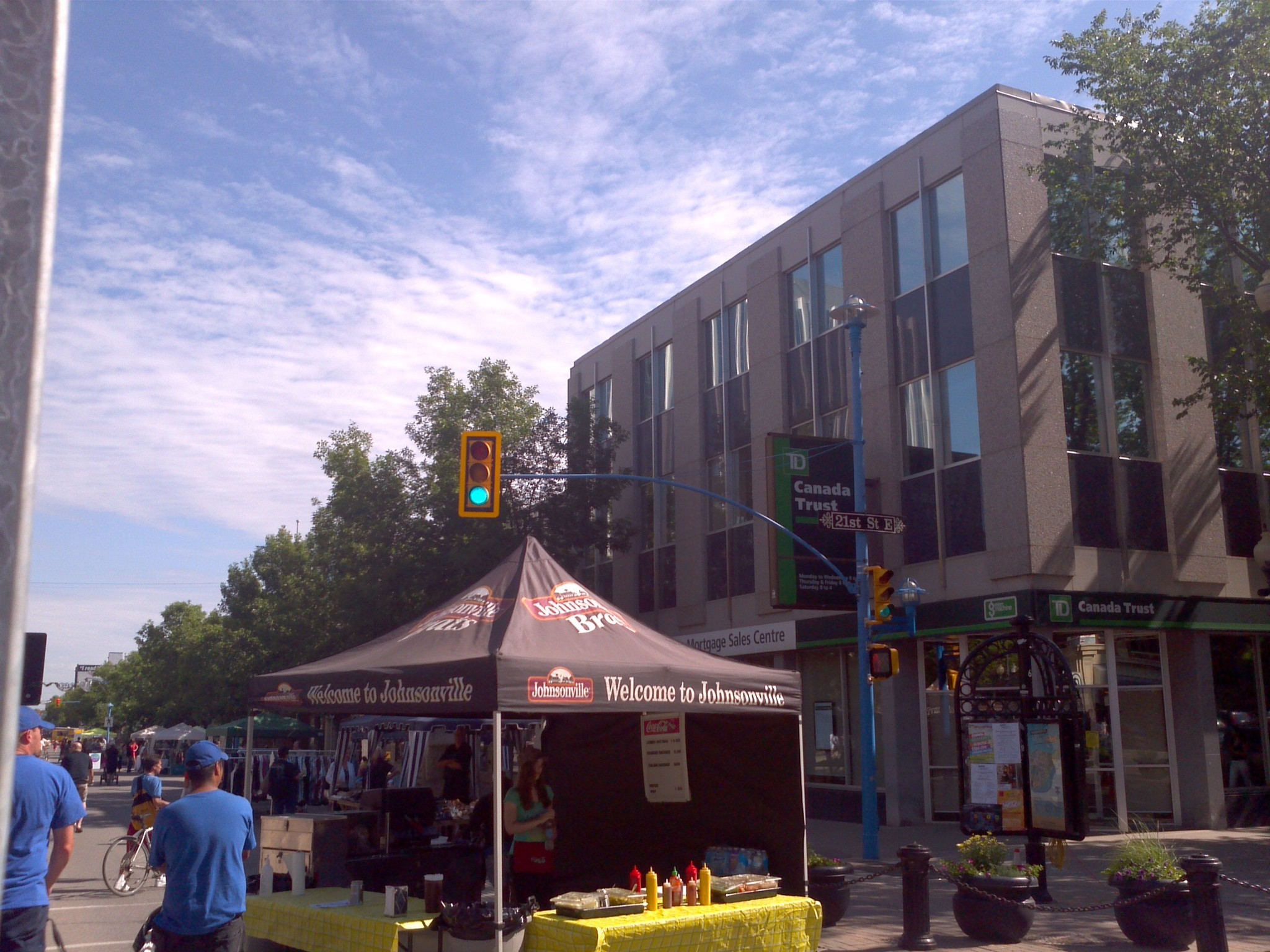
top-left (18, 705), bottom-right (55, 733)
top-left (184, 740), bottom-right (230, 771)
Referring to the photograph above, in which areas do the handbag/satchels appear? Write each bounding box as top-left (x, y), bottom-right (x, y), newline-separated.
top-left (130, 777), bottom-right (159, 831)
top-left (261, 764), bottom-right (273, 791)
top-left (512, 841), bottom-right (554, 875)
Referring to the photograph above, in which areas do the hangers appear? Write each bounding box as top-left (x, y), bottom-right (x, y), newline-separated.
top-left (224, 748), bottom-right (333, 761)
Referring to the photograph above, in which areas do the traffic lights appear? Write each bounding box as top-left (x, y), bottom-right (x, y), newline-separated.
top-left (459, 430), bottom-right (502, 520)
top-left (55, 698), bottom-right (61, 709)
top-left (873, 567), bottom-right (896, 622)
top-left (869, 646), bottom-right (900, 679)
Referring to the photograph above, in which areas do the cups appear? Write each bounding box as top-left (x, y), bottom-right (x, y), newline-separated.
top-left (424, 880), bottom-right (443, 913)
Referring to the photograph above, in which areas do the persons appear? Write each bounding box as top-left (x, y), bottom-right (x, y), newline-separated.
top-left (126, 738), bottom-right (139, 772)
top-left (105, 741), bottom-right (119, 779)
top-left (293, 740), bottom-right (303, 750)
top-left (61, 742), bottom-right (94, 832)
top-left (438, 726), bottom-right (472, 804)
top-left (268, 746), bottom-right (300, 814)
top-left (1226, 726), bottom-right (1253, 787)
top-left (362, 746), bottom-right (398, 789)
top-left (0, 706), bottom-right (86, 952)
top-left (502, 746), bottom-right (558, 909)
top-left (148, 740), bottom-right (257, 952)
top-left (58, 737), bottom-right (87, 762)
top-left (92, 736), bottom-right (107, 753)
top-left (114, 755), bottom-right (166, 891)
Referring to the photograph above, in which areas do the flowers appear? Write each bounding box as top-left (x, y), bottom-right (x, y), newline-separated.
top-left (1099, 807), bottom-right (1186, 883)
top-left (807, 846), bottom-right (847, 867)
top-left (938, 830), bottom-right (1044, 881)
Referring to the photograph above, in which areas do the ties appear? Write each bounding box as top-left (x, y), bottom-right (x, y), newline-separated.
top-left (344, 765), bottom-right (349, 788)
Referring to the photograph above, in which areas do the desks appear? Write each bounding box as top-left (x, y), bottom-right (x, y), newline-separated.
top-left (239, 885), bottom-right (443, 952)
top-left (523, 893), bottom-right (822, 952)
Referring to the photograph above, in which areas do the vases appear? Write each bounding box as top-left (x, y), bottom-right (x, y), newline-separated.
top-left (807, 862), bottom-right (855, 928)
top-left (952, 874), bottom-right (1039, 944)
top-left (1107, 876), bottom-right (1196, 952)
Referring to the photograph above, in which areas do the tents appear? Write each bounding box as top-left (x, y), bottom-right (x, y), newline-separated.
top-left (248, 536), bottom-right (809, 952)
top-left (206, 713), bottom-right (325, 750)
top-left (329, 715), bottom-right (541, 799)
top-left (82, 727), bottom-right (117, 738)
top-left (130, 724), bottom-right (206, 754)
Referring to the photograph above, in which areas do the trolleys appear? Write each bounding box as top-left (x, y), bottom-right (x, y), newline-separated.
top-left (99, 757), bottom-right (121, 786)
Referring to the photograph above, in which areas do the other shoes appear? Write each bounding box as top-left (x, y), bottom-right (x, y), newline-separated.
top-left (154, 875), bottom-right (167, 887)
top-left (111, 780), bottom-right (114, 785)
top-left (76, 829), bottom-right (82, 832)
top-left (107, 778), bottom-right (110, 785)
top-left (114, 880), bottom-right (130, 892)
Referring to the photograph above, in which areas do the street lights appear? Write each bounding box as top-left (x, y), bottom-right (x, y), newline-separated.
top-left (107, 702), bottom-right (114, 744)
top-left (830, 292), bottom-right (880, 868)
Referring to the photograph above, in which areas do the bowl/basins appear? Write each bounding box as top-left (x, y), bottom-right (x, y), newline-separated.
top-left (550, 888), bottom-right (647, 910)
top-left (696, 874), bottom-right (782, 894)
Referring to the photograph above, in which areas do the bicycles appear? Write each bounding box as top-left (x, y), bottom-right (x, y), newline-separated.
top-left (102, 813), bottom-right (165, 897)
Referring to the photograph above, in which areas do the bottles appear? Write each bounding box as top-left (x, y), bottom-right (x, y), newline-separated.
top-left (629, 865), bottom-right (641, 893)
top-left (663, 878), bottom-right (672, 908)
top-left (545, 822), bottom-right (553, 850)
top-left (687, 877), bottom-right (696, 906)
top-left (646, 867), bottom-right (657, 911)
top-left (669, 866), bottom-right (683, 906)
top-left (686, 861), bottom-right (697, 897)
top-left (700, 862), bottom-right (711, 905)
top-left (259, 855), bottom-right (273, 898)
top-left (705, 846), bottom-right (769, 877)
